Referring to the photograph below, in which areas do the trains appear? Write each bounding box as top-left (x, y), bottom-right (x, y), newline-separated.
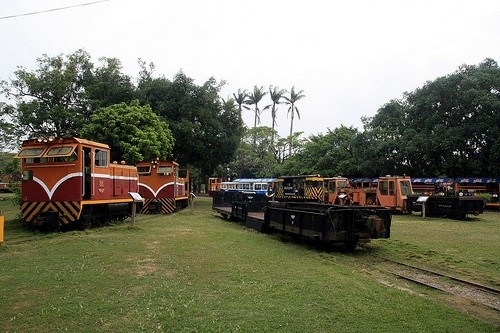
top-left (0, 131), bottom-right (198, 231)
top-left (206, 171), bottom-right (500, 252)
